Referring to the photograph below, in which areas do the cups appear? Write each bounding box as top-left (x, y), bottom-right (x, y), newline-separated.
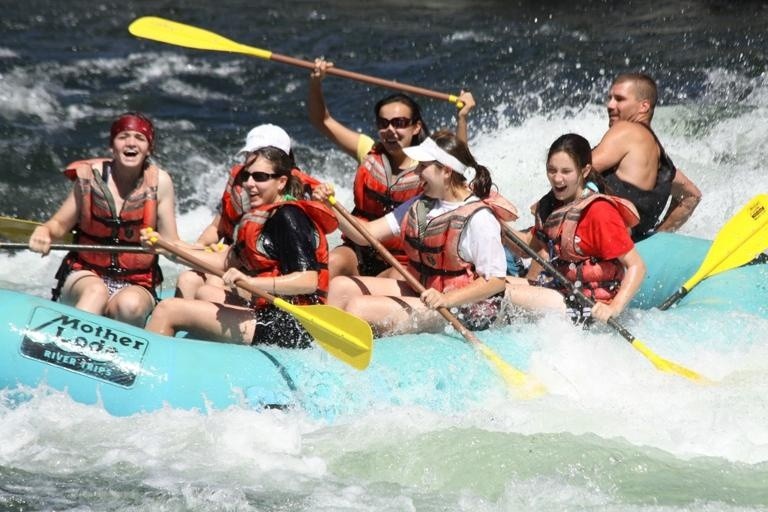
top-left (239, 170), bottom-right (286, 183)
top-left (375, 116), bottom-right (417, 129)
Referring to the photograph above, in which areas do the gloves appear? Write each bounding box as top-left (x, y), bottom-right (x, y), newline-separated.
top-left (0, 231), bottom-right (768, 417)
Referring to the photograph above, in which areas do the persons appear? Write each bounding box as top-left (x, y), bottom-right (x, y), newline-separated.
top-left (176, 124), bottom-right (323, 300)
top-left (307, 57), bottom-right (476, 241)
top-left (499, 133), bottom-right (647, 324)
top-left (138, 145), bottom-right (330, 349)
top-left (311, 129), bottom-right (508, 339)
top-left (29, 111), bottom-right (229, 326)
top-left (590, 72), bottom-right (703, 244)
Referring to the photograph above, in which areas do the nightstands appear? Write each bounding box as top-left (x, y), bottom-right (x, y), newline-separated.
top-left (494, 212), bottom-right (719, 388)
top-left (129, 17), bottom-right (465, 109)
top-left (0, 218), bottom-right (75, 244)
top-left (660, 194), bottom-right (768, 312)
top-left (147, 227), bottom-right (373, 370)
top-left (324, 183), bottom-right (547, 401)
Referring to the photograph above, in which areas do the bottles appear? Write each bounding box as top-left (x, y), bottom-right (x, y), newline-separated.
top-left (236, 123), bottom-right (292, 155)
top-left (403, 137), bottom-right (477, 183)
